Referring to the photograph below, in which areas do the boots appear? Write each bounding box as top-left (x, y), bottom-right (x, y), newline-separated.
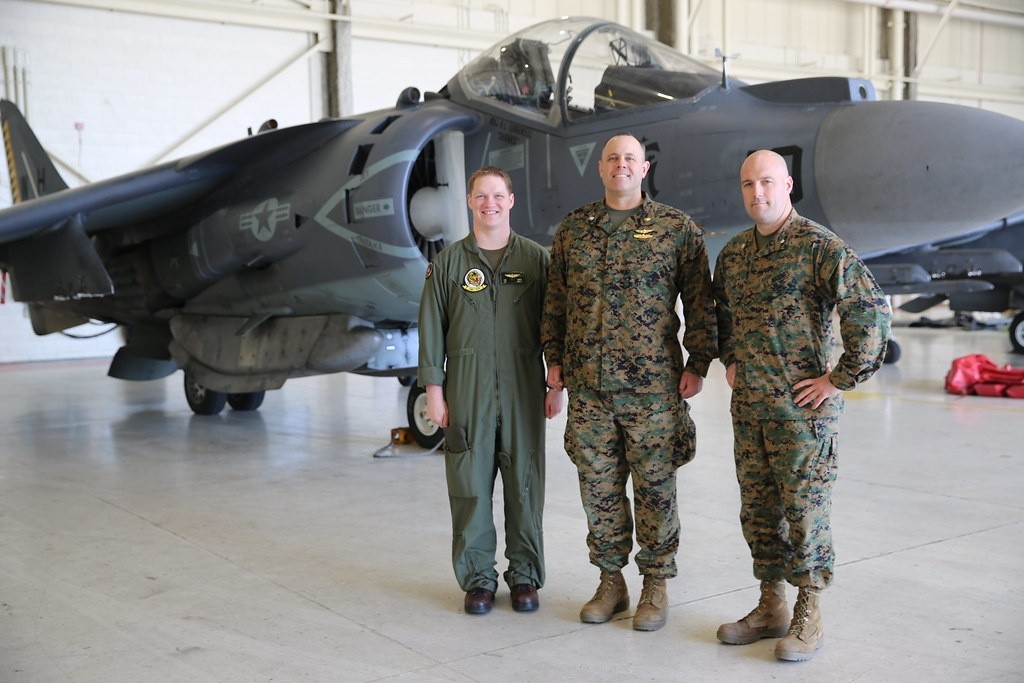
top-left (717, 581), bottom-right (791, 645)
top-left (775, 588), bottom-right (824, 661)
top-left (580, 571), bottom-right (629, 623)
top-left (634, 576), bottom-right (668, 630)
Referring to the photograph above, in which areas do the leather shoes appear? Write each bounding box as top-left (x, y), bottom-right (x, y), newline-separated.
top-left (510, 584), bottom-right (539, 611)
top-left (464, 587), bottom-right (495, 614)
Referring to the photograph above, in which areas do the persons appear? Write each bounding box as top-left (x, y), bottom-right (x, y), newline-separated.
top-left (717, 150), bottom-right (890, 660)
top-left (540, 132), bottom-right (715, 630)
top-left (417, 167), bottom-right (564, 614)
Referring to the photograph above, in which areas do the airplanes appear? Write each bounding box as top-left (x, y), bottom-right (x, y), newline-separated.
top-left (0, 17), bottom-right (1024, 431)
